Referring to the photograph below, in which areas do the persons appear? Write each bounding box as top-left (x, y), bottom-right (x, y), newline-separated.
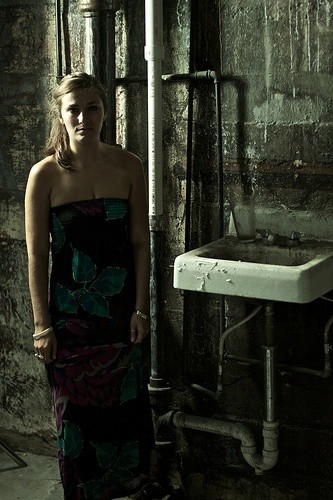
top-left (21, 72), bottom-right (152, 500)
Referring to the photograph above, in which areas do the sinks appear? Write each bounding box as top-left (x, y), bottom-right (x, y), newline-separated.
top-left (173, 233), bottom-right (333, 302)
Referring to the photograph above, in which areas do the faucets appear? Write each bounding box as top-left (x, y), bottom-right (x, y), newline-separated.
top-left (290, 231), bottom-right (302, 240)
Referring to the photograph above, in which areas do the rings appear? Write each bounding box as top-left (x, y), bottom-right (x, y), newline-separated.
top-left (34, 353), bottom-right (45, 360)
top-left (130, 329), bottom-right (135, 337)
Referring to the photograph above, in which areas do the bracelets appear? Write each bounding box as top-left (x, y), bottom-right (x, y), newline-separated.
top-left (33, 325), bottom-right (53, 340)
top-left (133, 307), bottom-right (152, 322)
